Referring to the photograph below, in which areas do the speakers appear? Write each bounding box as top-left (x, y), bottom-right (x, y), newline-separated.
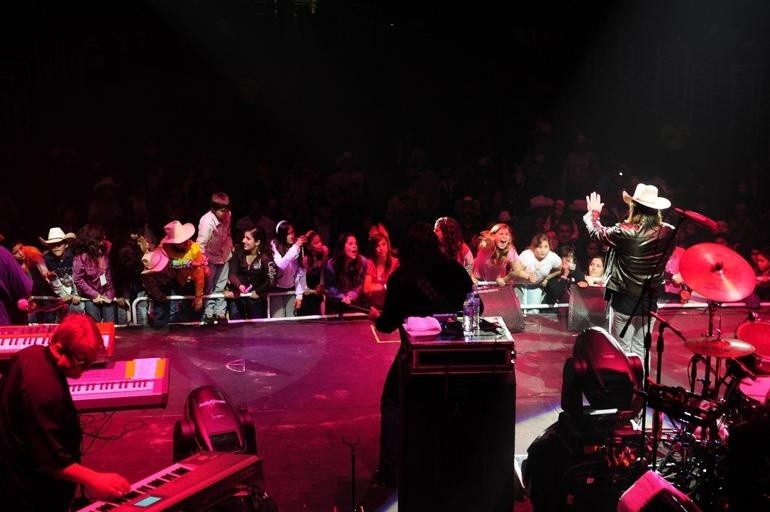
top-left (397, 351), bottom-right (516, 512)
top-left (568, 285), bottom-right (609, 332)
top-left (476, 285), bottom-right (525, 333)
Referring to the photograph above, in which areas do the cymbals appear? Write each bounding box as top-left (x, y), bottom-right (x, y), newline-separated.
top-left (679, 244), bottom-right (756, 302)
top-left (686, 336), bottom-right (754, 358)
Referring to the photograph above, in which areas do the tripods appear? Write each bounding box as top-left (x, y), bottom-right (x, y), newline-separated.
top-left (619, 221), bottom-right (741, 512)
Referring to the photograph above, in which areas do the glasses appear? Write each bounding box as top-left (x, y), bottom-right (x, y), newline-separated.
top-left (74, 353), bottom-right (89, 370)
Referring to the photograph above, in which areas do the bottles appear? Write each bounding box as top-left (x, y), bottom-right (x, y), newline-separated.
top-left (462, 290), bottom-right (480, 336)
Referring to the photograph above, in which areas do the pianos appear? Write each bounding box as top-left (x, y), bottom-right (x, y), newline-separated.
top-left (80, 451), bottom-right (262, 510)
top-left (64, 358), bottom-right (169, 408)
top-left (1, 324), bottom-right (115, 370)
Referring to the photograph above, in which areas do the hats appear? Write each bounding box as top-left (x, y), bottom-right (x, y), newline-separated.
top-left (569, 199), bottom-right (588, 213)
top-left (139, 247), bottom-right (170, 274)
top-left (160, 219), bottom-right (196, 244)
top-left (38, 226), bottom-right (77, 247)
top-left (623, 183), bottom-right (671, 210)
top-left (528, 195), bottom-right (552, 211)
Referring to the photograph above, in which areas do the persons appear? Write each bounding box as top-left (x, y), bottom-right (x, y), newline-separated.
top-left (583, 179), bottom-right (676, 374)
top-left (1, 140), bottom-right (769, 335)
top-left (0, 309), bottom-right (132, 512)
top-left (359, 218), bottom-right (487, 496)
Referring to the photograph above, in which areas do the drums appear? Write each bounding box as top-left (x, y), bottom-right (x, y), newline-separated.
top-left (734, 321), bottom-right (770, 376)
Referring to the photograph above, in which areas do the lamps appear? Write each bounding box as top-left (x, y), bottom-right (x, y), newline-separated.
top-left (171, 380), bottom-right (259, 467)
top-left (559, 324), bottom-right (647, 426)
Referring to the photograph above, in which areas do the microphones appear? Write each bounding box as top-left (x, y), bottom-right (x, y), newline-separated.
top-left (673, 207), bottom-right (729, 234)
